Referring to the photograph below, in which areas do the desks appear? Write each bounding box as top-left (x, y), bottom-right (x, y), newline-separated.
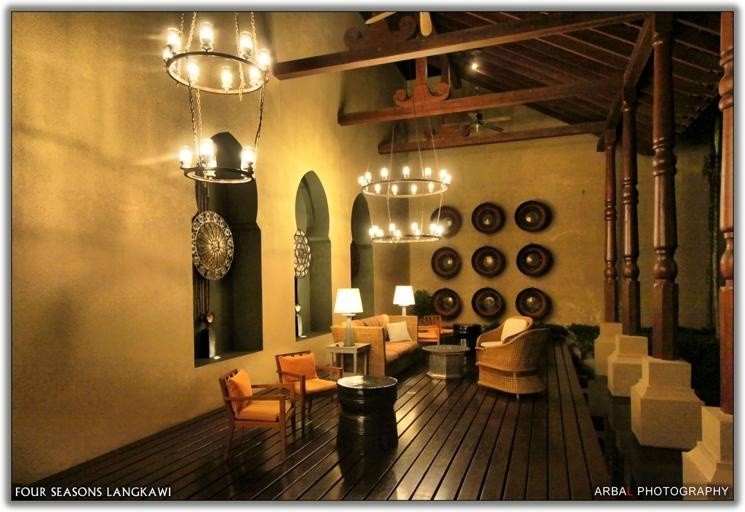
top-left (326, 340), bottom-right (371, 377)
top-left (334, 374), bottom-right (401, 457)
top-left (416, 322), bottom-right (442, 350)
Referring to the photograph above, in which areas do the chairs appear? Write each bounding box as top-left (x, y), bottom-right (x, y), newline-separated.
top-left (476, 312), bottom-right (536, 364)
top-left (476, 325), bottom-right (554, 403)
top-left (217, 366), bottom-right (299, 467)
top-left (273, 345), bottom-right (343, 437)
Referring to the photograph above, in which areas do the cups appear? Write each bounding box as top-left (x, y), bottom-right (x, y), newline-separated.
top-left (460, 338), bottom-right (467, 350)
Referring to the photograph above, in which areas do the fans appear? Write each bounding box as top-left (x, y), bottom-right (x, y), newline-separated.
top-left (442, 75), bottom-right (514, 143)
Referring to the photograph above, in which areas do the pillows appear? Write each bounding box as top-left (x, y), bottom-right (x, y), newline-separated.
top-left (499, 315), bottom-right (530, 342)
top-left (277, 351), bottom-right (318, 385)
top-left (382, 320), bottom-right (416, 343)
top-left (223, 367), bottom-right (254, 415)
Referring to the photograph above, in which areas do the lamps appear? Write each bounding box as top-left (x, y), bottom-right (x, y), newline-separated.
top-left (158, 11), bottom-right (275, 184)
top-left (355, 91), bottom-right (454, 247)
top-left (392, 281), bottom-right (416, 319)
top-left (332, 286), bottom-right (366, 345)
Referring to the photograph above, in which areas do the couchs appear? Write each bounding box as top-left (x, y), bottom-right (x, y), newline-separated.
top-left (326, 314), bottom-right (422, 377)
